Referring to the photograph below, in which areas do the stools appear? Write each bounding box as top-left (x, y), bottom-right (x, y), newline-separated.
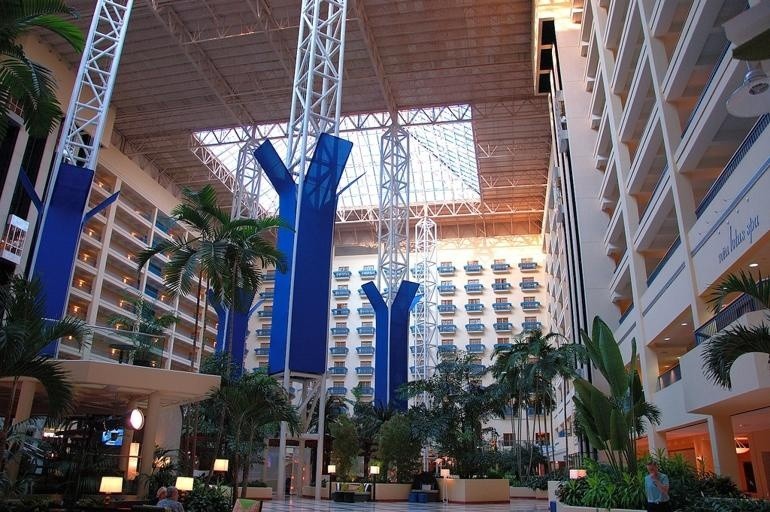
top-left (549, 500), bottom-right (557, 512)
top-left (332, 472), bottom-right (440, 503)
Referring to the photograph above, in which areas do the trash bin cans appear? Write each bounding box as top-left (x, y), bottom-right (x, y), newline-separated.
top-left (334, 492), bottom-right (355, 503)
top-left (408, 493), bottom-right (428, 503)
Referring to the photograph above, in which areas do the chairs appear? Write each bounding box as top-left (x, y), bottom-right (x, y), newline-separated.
top-left (232, 496), bottom-right (264, 511)
top-left (131, 503), bottom-right (166, 512)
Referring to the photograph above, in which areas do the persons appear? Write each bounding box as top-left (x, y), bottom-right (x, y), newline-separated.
top-left (644, 461), bottom-right (670, 512)
top-left (157, 486), bottom-right (167, 500)
top-left (156, 486), bottom-right (185, 512)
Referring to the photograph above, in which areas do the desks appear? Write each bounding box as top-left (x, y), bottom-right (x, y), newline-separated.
top-left (109, 342), bottom-right (136, 364)
top-left (90, 493), bottom-right (153, 512)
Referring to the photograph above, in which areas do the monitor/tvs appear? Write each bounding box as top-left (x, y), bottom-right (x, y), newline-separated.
top-left (102, 425), bottom-right (123, 445)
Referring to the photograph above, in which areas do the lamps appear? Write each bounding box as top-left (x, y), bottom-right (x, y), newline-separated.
top-left (326, 464), bottom-right (338, 500)
top-left (92, 393), bottom-right (145, 437)
top-left (440, 468), bottom-right (452, 503)
top-left (726, 53), bottom-right (770, 122)
top-left (173, 474), bottom-right (195, 502)
top-left (213, 457), bottom-right (230, 482)
top-left (370, 464), bottom-right (381, 499)
top-left (98, 475), bottom-right (125, 502)
